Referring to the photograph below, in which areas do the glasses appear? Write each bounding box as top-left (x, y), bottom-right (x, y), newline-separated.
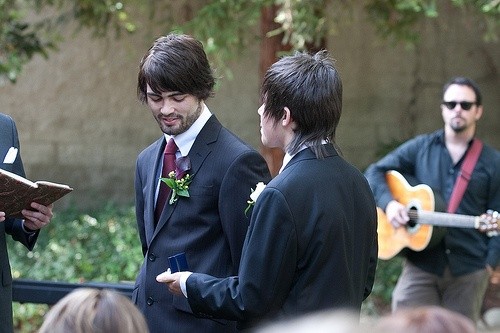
top-left (442, 101), bottom-right (476, 110)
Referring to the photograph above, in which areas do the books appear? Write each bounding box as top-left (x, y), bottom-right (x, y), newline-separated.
top-left (0, 167), bottom-right (74, 219)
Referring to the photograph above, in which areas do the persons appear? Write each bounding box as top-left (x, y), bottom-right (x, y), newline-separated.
top-left (263, 305), bottom-right (497, 333)
top-left (156, 51), bottom-right (378, 333)
top-left (39, 286), bottom-right (148, 333)
top-left (133, 35), bottom-right (272, 333)
top-left (0, 114), bottom-right (53, 333)
top-left (365, 77), bottom-right (500, 322)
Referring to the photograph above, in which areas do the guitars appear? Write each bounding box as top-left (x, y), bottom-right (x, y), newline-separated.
top-left (377, 169), bottom-right (500, 261)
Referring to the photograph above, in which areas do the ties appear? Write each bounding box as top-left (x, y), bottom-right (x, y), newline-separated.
top-left (154, 137), bottom-right (179, 233)
